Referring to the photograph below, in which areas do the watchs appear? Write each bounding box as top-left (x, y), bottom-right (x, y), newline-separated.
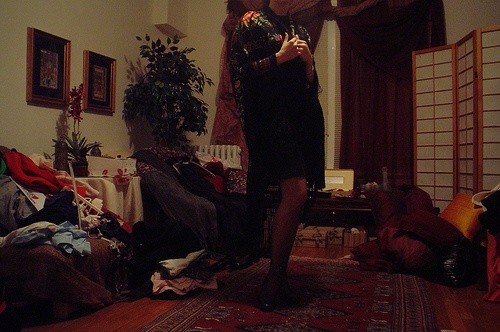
top-left (268, 53), bottom-right (277, 66)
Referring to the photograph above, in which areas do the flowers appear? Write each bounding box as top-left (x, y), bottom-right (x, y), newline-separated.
top-left (51, 83), bottom-right (104, 160)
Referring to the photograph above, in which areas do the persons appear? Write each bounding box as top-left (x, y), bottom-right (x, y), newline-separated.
top-left (225, 0), bottom-right (325, 311)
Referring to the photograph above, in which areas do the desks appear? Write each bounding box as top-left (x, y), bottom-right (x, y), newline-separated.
top-left (63, 174), bottom-right (145, 247)
top-left (306, 199), bottom-right (380, 252)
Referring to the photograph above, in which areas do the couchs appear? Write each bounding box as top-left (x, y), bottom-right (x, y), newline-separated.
top-left (0, 145), bottom-right (134, 319)
top-left (130, 148), bottom-right (260, 258)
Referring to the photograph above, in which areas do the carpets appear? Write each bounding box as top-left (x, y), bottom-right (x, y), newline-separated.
top-left (137, 255), bottom-right (439, 332)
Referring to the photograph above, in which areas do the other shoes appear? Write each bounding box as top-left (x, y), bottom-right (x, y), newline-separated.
top-left (254, 280), bottom-right (300, 312)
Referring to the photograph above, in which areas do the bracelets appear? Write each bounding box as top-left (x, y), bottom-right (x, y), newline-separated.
top-left (255, 57), bottom-right (262, 72)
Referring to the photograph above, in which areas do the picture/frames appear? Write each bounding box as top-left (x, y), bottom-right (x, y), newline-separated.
top-left (26, 27), bottom-right (71, 110)
top-left (82, 50), bottom-right (116, 113)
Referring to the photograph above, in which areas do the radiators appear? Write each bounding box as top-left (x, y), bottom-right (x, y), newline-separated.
top-left (197, 144), bottom-right (241, 161)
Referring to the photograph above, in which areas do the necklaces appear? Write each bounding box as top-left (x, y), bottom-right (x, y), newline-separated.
top-left (265, 7), bottom-right (294, 37)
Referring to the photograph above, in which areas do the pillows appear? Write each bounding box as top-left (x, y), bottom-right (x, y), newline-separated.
top-left (350, 184), bottom-right (484, 274)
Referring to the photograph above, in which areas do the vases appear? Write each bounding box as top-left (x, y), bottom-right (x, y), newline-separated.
top-left (69, 159), bottom-right (88, 177)
